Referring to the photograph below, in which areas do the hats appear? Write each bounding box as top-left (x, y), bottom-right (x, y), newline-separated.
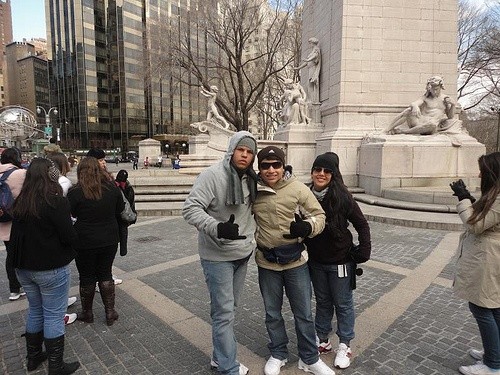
top-left (257, 145), bottom-right (285, 166)
top-left (87, 147), bottom-right (106, 159)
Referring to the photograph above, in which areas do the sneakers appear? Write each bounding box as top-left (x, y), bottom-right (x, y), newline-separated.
top-left (298, 357), bottom-right (335, 375)
top-left (315, 337), bottom-right (332, 355)
top-left (263, 355), bottom-right (288, 375)
top-left (334, 342), bottom-right (353, 369)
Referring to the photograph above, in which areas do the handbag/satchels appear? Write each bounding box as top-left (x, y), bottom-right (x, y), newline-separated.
top-left (261, 242), bottom-right (306, 266)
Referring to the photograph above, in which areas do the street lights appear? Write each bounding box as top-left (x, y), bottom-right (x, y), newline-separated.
top-left (36, 106), bottom-right (59, 145)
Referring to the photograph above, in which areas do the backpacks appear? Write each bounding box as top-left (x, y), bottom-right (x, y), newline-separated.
top-left (0, 165), bottom-right (17, 212)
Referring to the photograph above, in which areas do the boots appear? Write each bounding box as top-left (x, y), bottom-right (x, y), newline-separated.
top-left (98, 280), bottom-right (118, 326)
top-left (77, 281), bottom-right (96, 323)
top-left (43, 334), bottom-right (80, 375)
top-left (20, 332), bottom-right (48, 372)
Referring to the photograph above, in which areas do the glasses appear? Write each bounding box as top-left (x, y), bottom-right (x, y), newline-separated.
top-left (260, 161), bottom-right (282, 169)
top-left (314, 166), bottom-right (331, 173)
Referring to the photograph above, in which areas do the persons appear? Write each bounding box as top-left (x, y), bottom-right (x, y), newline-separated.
top-left (200, 85), bottom-right (231, 129)
top-left (182, 130), bottom-right (259, 375)
top-left (251, 146), bottom-right (335, 375)
top-left (279, 37), bottom-right (322, 124)
top-left (449, 152), bottom-right (500, 375)
top-left (373, 76), bottom-right (455, 136)
top-left (0, 142), bottom-right (137, 375)
top-left (306, 152), bottom-right (371, 369)
top-left (132, 155), bottom-right (180, 169)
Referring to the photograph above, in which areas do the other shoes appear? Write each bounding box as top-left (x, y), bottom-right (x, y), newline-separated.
top-left (459, 362), bottom-right (500, 375)
top-left (64, 312), bottom-right (77, 325)
top-left (210, 360), bottom-right (249, 375)
top-left (9, 286), bottom-right (26, 300)
top-left (68, 296), bottom-right (77, 306)
top-left (470, 349), bottom-right (483, 360)
top-left (112, 275), bottom-right (122, 285)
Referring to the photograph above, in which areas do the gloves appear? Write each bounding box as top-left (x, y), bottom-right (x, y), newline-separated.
top-left (449, 178), bottom-right (477, 202)
top-left (283, 213), bottom-right (311, 239)
top-left (217, 214), bottom-right (247, 240)
top-left (117, 170), bottom-right (128, 182)
top-left (351, 250), bottom-right (370, 264)
